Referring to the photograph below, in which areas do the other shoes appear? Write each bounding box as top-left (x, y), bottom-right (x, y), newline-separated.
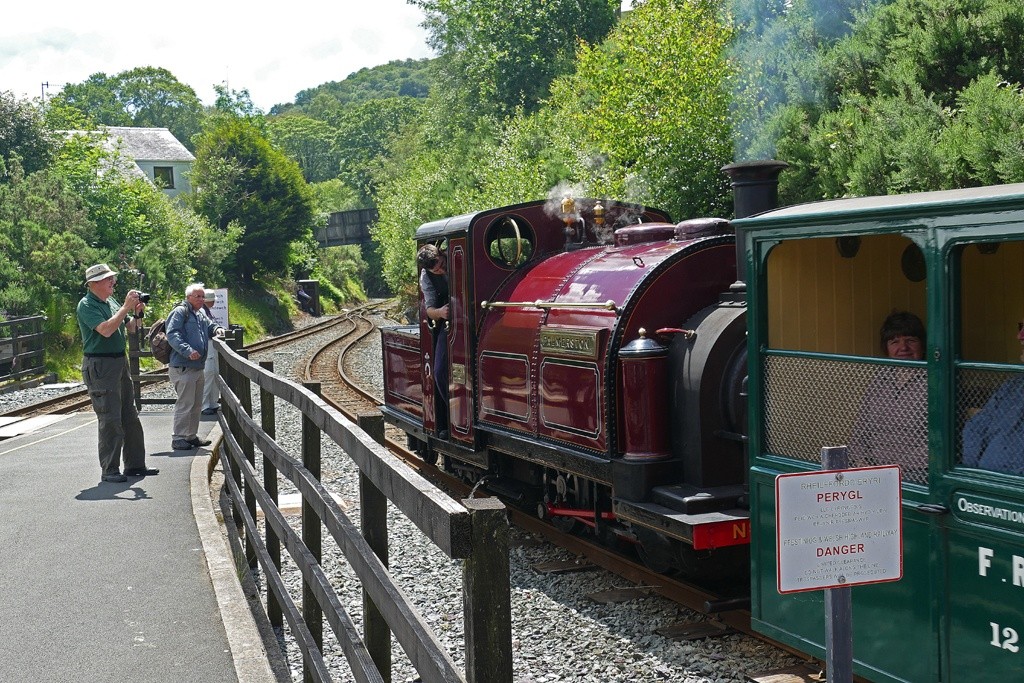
top-left (201, 408), bottom-right (218, 415)
top-left (439, 429), bottom-right (449, 439)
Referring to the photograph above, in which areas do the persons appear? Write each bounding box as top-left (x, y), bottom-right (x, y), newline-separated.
top-left (199, 289), bottom-right (220, 414)
top-left (77, 264), bottom-right (159, 482)
top-left (165, 284), bottom-right (224, 450)
top-left (853, 310), bottom-right (1024, 475)
top-left (417, 243), bottom-right (449, 440)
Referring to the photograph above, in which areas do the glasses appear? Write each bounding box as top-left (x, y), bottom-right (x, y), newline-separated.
top-left (190, 294), bottom-right (205, 298)
top-left (1018, 322), bottom-right (1024, 331)
top-left (107, 276), bottom-right (113, 283)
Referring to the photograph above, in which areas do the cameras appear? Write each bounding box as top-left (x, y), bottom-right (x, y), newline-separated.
top-left (136, 291), bottom-right (150, 304)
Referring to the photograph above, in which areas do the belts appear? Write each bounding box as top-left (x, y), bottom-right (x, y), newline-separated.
top-left (84, 351), bottom-right (125, 357)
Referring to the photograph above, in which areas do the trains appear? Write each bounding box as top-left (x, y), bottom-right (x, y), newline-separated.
top-left (378, 160), bottom-right (1024, 683)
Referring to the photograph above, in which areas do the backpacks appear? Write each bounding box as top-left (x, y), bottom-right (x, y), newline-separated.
top-left (144, 304), bottom-right (189, 364)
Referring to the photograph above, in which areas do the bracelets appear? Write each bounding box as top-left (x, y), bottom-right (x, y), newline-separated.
top-left (133, 315), bottom-right (139, 319)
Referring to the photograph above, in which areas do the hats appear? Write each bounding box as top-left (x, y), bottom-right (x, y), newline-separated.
top-left (84, 264), bottom-right (118, 288)
top-left (204, 289), bottom-right (218, 301)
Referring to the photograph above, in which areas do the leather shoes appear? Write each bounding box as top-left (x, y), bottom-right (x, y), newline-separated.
top-left (172, 439), bottom-right (191, 450)
top-left (186, 436), bottom-right (212, 446)
top-left (123, 467), bottom-right (159, 476)
top-left (101, 474), bottom-right (127, 482)
